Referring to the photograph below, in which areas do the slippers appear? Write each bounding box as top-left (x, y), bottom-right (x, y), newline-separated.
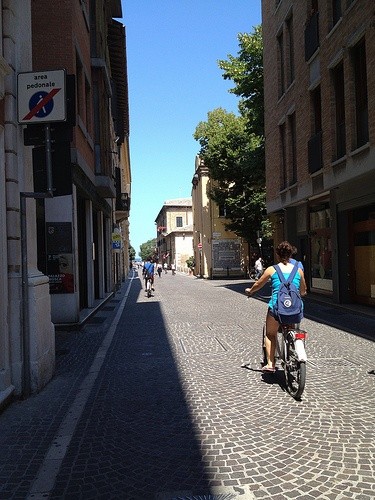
top-left (262, 365), bottom-right (276, 372)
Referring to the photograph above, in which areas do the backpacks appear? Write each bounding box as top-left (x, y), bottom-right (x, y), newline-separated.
top-left (273, 264), bottom-right (303, 324)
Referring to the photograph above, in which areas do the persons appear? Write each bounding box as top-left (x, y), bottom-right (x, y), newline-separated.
top-left (288, 246), bottom-right (304, 272)
top-left (142, 258), bottom-right (155, 292)
top-left (245, 241), bottom-right (306, 371)
top-left (157, 262), bottom-right (176, 277)
top-left (255, 258), bottom-right (263, 281)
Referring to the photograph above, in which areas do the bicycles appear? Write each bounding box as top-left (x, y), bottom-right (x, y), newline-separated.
top-left (142, 273), bottom-right (156, 298)
top-left (246, 292), bottom-right (308, 400)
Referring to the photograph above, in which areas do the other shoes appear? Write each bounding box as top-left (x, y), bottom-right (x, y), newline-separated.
top-left (151, 288), bottom-right (155, 291)
